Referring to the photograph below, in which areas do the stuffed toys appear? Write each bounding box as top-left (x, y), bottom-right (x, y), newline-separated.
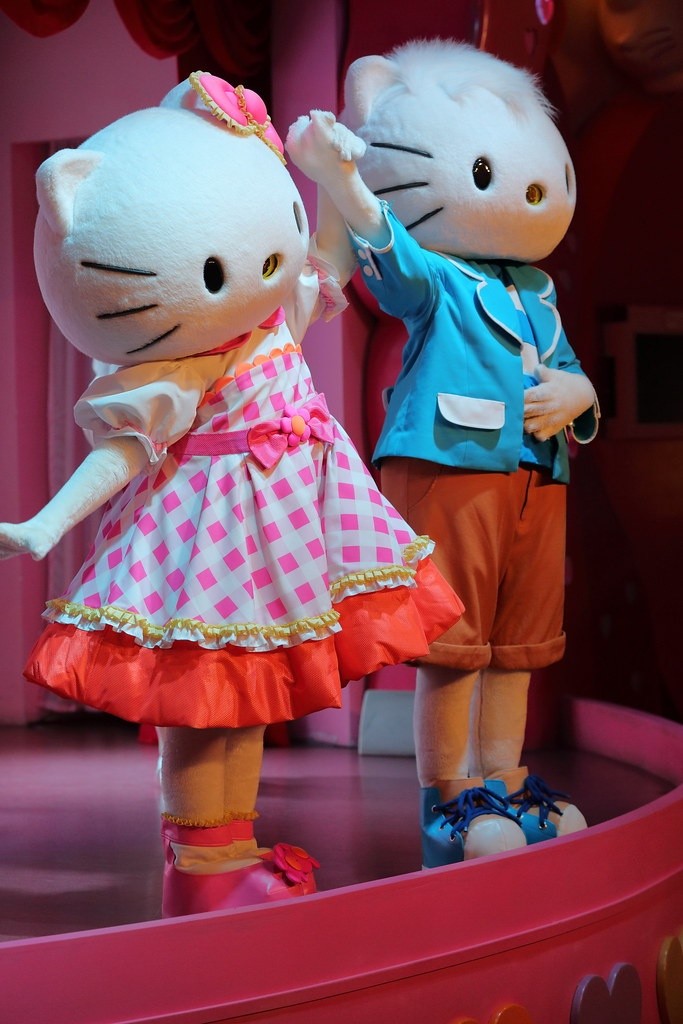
top-left (0, 69), bottom-right (367, 919)
top-left (284, 39), bottom-right (601, 869)
